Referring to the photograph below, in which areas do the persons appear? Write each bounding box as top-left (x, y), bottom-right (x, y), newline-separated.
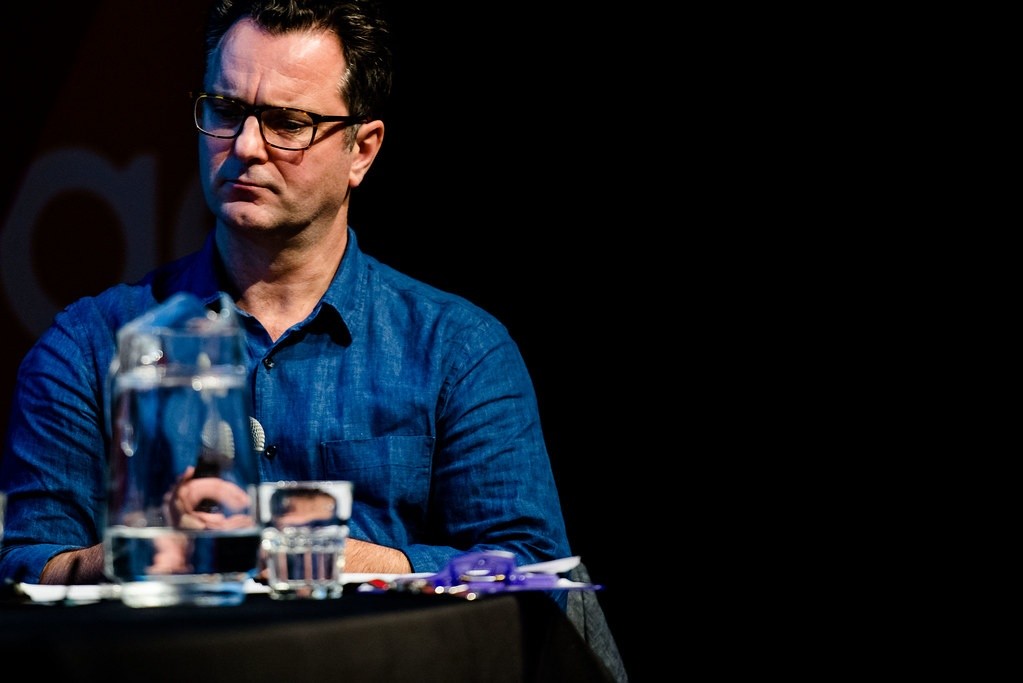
top-left (0, 0), bottom-right (574, 624)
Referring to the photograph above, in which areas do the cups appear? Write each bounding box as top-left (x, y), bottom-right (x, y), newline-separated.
top-left (104, 329), bottom-right (260, 606)
top-left (259, 481), bottom-right (353, 601)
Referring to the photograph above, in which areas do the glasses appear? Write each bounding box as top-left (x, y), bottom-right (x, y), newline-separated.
top-left (189, 91), bottom-right (373, 151)
